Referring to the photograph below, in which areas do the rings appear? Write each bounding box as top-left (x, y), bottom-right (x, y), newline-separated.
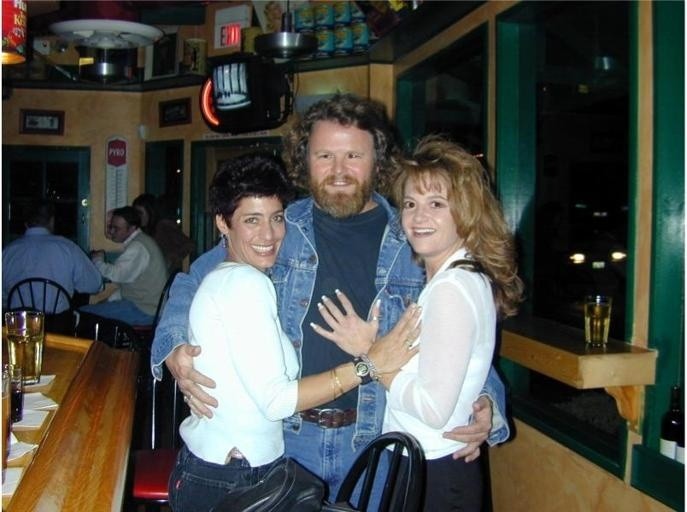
top-left (370, 316), bottom-right (379, 321)
top-left (183, 396), bottom-right (189, 403)
top-left (187, 393), bottom-right (192, 400)
top-left (405, 339), bottom-right (413, 348)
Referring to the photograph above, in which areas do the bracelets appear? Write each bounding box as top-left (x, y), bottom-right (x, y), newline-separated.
top-left (334, 367), bottom-right (344, 394)
top-left (330, 367), bottom-right (335, 400)
top-left (360, 354), bottom-right (380, 381)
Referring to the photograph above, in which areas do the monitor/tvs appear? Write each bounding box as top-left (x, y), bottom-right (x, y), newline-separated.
top-left (203, 53), bottom-right (281, 128)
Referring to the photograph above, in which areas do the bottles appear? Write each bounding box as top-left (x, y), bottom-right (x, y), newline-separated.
top-left (659, 384), bottom-right (684, 464)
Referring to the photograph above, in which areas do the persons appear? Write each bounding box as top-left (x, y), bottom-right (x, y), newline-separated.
top-left (169, 152), bottom-right (423, 512)
top-left (132, 193), bottom-right (194, 273)
top-left (150, 91), bottom-right (511, 512)
top-left (2, 198), bottom-right (102, 336)
top-left (309, 134), bottom-right (526, 512)
top-left (75, 206), bottom-right (168, 326)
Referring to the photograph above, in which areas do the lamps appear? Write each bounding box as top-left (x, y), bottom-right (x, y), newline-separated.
top-left (2, 0), bottom-right (28, 68)
top-left (49, 17), bottom-right (164, 86)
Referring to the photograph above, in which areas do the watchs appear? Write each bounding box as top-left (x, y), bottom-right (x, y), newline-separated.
top-left (352, 357), bottom-right (372, 385)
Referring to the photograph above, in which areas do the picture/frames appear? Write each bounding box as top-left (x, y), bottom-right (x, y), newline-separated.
top-left (156, 97), bottom-right (193, 128)
top-left (17, 108), bottom-right (67, 137)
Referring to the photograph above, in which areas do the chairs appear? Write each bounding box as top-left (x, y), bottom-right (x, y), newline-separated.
top-left (334, 431), bottom-right (427, 512)
top-left (79, 271), bottom-right (184, 349)
top-left (5, 274), bottom-right (77, 337)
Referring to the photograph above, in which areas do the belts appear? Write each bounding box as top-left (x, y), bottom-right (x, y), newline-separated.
top-left (303, 408), bottom-right (356, 428)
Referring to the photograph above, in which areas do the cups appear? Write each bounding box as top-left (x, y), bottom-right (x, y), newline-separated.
top-left (2, 362), bottom-right (22, 471)
top-left (581, 293), bottom-right (610, 346)
top-left (4, 311), bottom-right (43, 384)
